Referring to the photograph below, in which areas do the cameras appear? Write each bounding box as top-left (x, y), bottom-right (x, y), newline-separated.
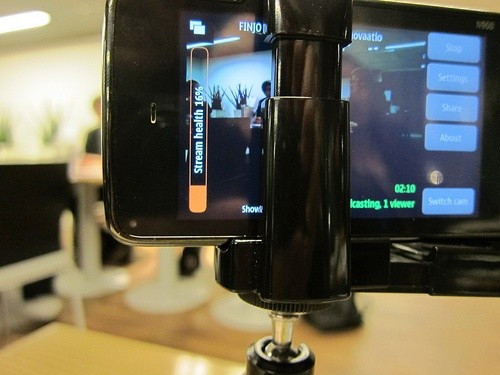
top-left (99, 0), bottom-right (499, 317)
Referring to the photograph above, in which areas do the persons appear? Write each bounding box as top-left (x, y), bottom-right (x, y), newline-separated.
top-left (243, 80), bottom-right (271, 157)
top-left (83, 94), bottom-right (101, 157)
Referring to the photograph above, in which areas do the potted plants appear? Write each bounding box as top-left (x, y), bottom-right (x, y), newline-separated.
top-left (223, 83), bottom-right (253, 117)
top-left (204, 83), bottom-right (225, 110)
